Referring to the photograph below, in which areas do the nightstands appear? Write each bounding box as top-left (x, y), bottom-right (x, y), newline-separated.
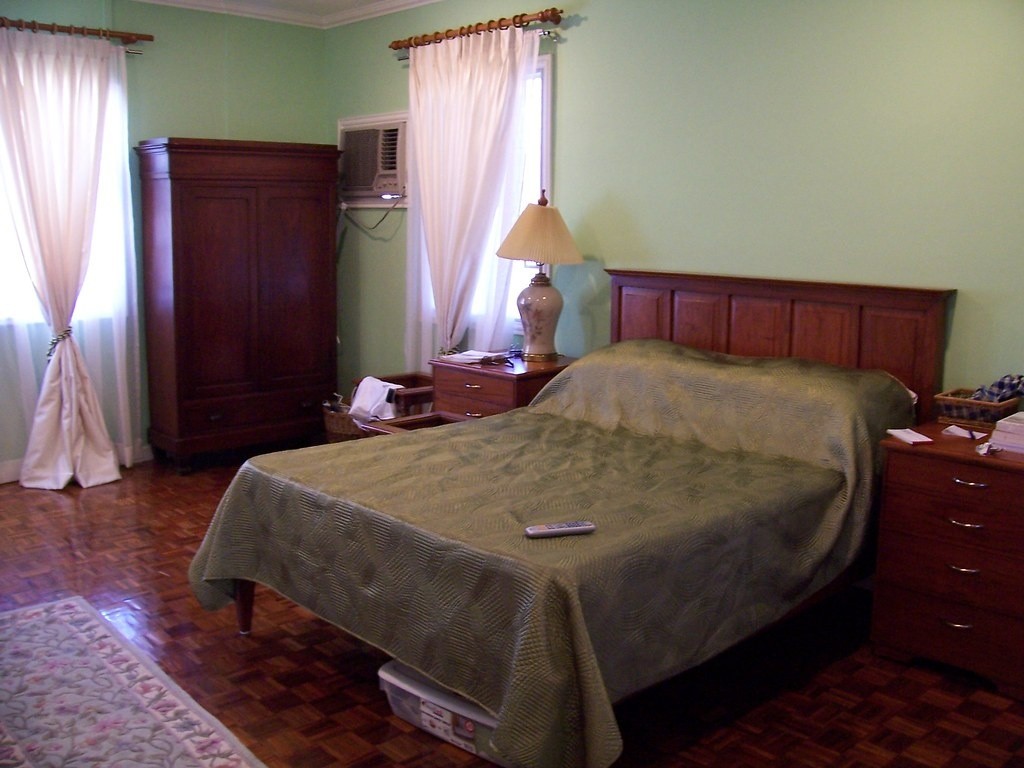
top-left (428, 350), bottom-right (580, 418)
top-left (871, 420), bottom-right (1024, 702)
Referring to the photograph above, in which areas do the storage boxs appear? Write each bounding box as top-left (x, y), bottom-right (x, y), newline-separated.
top-left (378, 660), bottom-right (503, 766)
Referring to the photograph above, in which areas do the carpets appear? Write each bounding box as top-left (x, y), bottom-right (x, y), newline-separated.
top-left (0, 595), bottom-right (270, 768)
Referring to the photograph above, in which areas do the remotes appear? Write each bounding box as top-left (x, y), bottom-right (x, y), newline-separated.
top-left (524, 520), bottom-right (597, 538)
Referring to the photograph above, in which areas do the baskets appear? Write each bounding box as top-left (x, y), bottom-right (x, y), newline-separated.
top-left (933, 388), bottom-right (1021, 433)
top-left (325, 398), bottom-right (369, 444)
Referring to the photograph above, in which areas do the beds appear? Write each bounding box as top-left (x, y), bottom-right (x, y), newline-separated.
top-left (188, 267), bottom-right (957, 767)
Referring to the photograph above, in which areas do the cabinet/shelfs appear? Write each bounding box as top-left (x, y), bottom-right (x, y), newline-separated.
top-left (134, 137), bottom-right (346, 474)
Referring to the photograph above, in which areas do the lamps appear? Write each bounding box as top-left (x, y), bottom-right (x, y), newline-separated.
top-left (494, 190), bottom-right (583, 361)
